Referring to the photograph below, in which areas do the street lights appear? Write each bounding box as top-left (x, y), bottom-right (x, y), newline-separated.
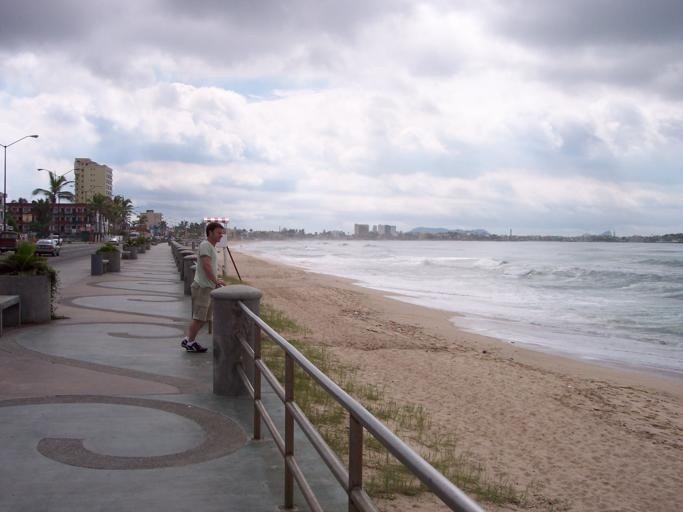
top-left (37, 165), bottom-right (83, 235)
top-left (0, 135), bottom-right (39, 231)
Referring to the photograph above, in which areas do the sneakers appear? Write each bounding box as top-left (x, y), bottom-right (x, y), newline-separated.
top-left (181, 336), bottom-right (208, 353)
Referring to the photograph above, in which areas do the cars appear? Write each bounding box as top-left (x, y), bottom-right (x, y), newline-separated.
top-left (153, 234), bottom-right (162, 241)
top-left (107, 237), bottom-right (119, 245)
top-left (127, 232), bottom-right (140, 243)
top-left (0, 230), bottom-right (18, 252)
top-left (34, 234), bottom-right (63, 257)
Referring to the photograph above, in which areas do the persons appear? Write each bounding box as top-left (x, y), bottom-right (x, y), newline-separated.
top-left (181, 222), bottom-right (226, 352)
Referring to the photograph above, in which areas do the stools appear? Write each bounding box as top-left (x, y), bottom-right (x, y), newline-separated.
top-left (0, 295), bottom-right (21, 334)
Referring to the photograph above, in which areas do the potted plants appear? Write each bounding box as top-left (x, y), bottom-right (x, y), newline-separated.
top-left (0, 239), bottom-right (61, 323)
top-left (96, 245), bottom-right (122, 273)
top-left (120, 237), bottom-right (149, 258)
